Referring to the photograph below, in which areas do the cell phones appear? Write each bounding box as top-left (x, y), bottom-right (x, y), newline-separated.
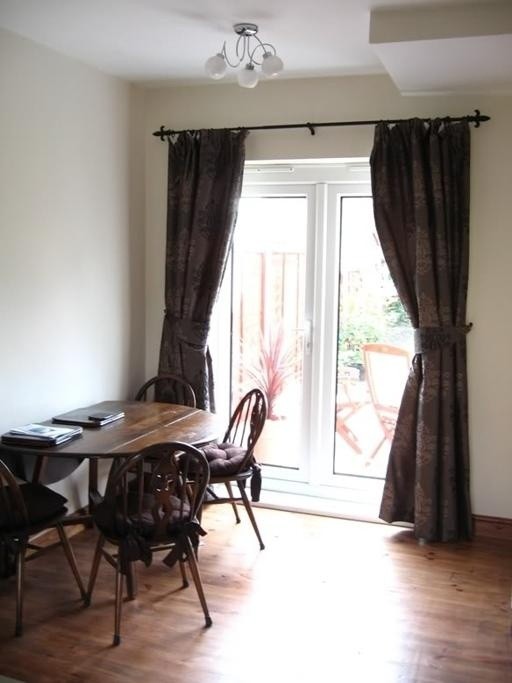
top-left (88, 412), bottom-right (114, 419)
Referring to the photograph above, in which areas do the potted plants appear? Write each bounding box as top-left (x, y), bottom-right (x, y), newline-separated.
top-left (239, 325), bottom-right (300, 456)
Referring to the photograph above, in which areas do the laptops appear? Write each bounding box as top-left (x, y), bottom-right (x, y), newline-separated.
top-left (52, 408), bottom-right (125, 427)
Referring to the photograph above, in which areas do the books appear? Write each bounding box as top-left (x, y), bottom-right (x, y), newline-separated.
top-left (1, 408), bottom-right (125, 447)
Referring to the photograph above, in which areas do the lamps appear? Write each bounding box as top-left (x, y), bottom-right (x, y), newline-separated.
top-left (205, 23), bottom-right (283, 88)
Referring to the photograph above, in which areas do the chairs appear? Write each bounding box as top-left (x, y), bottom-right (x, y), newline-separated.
top-left (0, 459), bottom-right (86, 638)
top-left (336, 343), bottom-right (413, 464)
top-left (105, 374), bottom-right (197, 500)
top-left (87, 441), bottom-right (213, 646)
top-left (173, 388), bottom-right (267, 561)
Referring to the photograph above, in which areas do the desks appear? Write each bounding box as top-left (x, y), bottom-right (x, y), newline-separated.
top-left (0, 400), bottom-right (224, 529)
